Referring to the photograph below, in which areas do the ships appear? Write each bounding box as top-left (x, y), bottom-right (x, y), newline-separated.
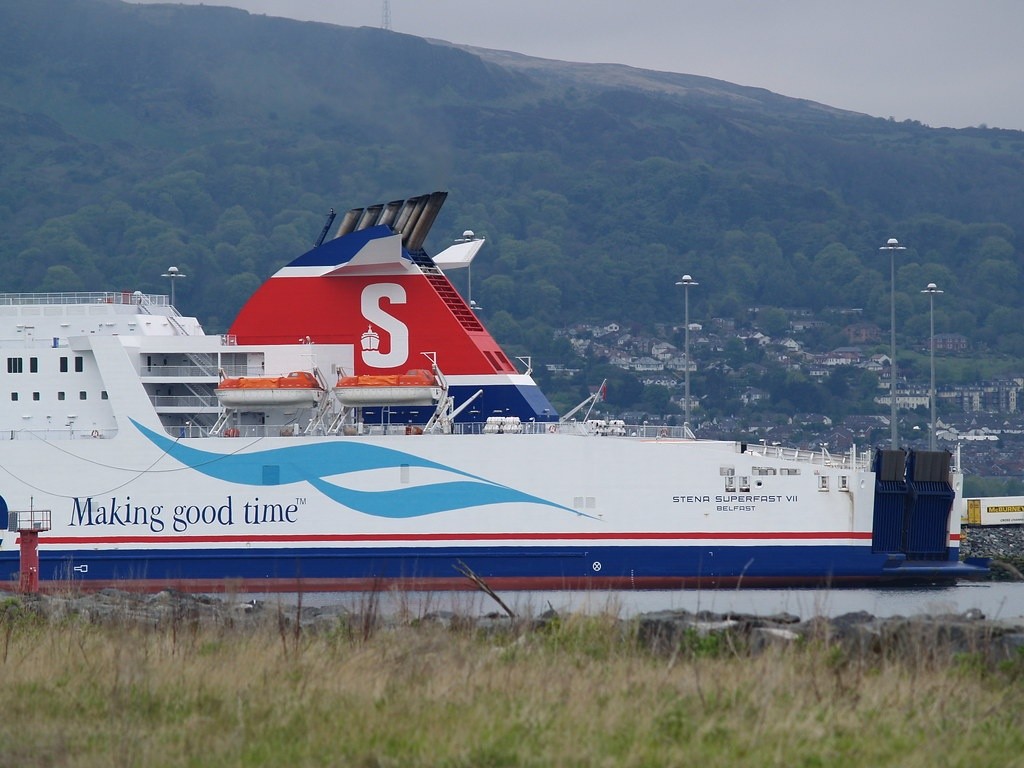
top-left (0, 191), bottom-right (994, 583)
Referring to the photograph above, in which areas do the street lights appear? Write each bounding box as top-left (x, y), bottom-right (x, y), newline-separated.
top-left (920, 281), bottom-right (944, 451)
top-left (878, 238), bottom-right (906, 450)
top-left (160, 266), bottom-right (186, 309)
top-left (454, 228), bottom-right (480, 309)
top-left (674, 274), bottom-right (699, 428)
